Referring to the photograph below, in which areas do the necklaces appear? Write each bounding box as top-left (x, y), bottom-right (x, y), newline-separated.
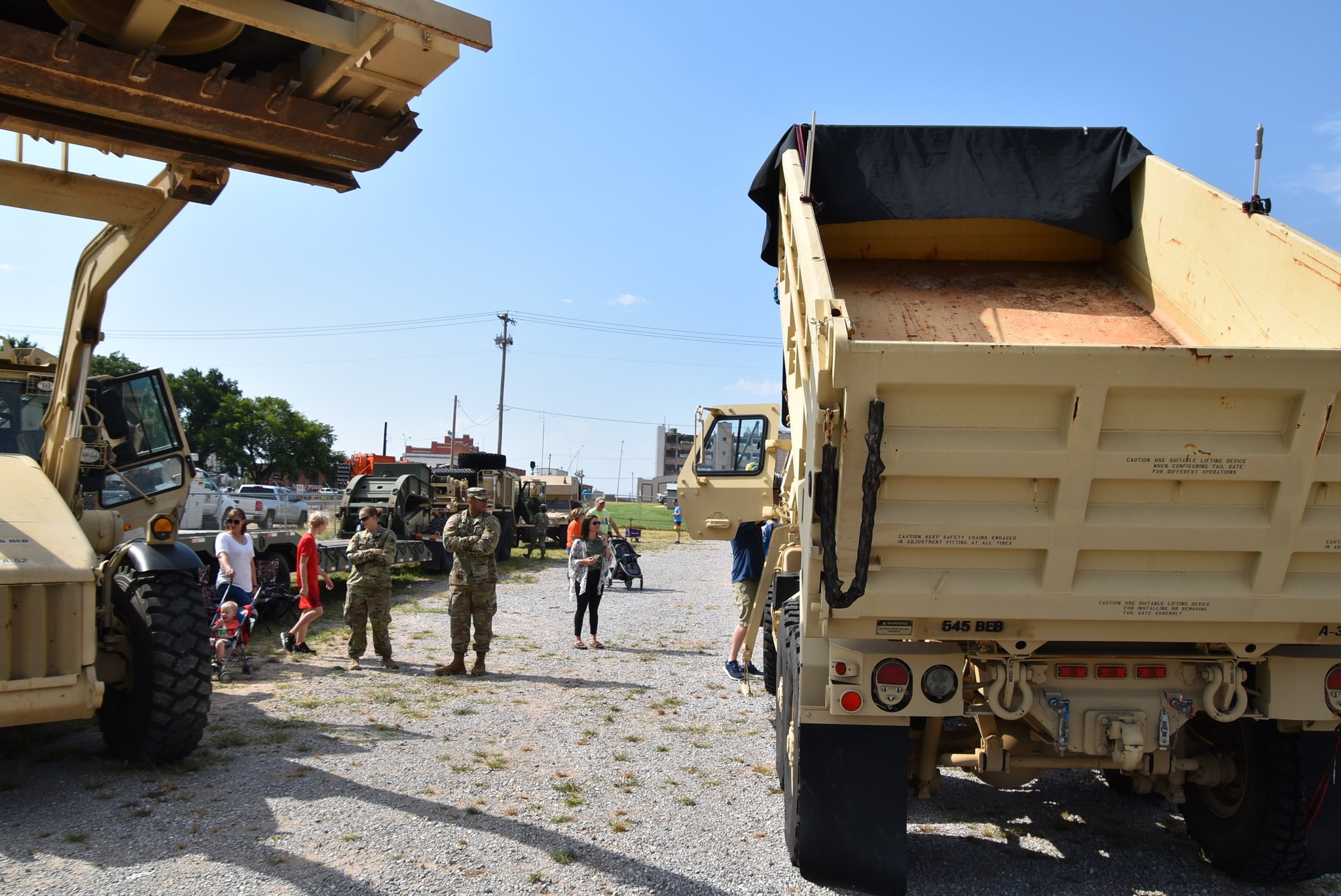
top-left (234, 534), bottom-right (244, 542)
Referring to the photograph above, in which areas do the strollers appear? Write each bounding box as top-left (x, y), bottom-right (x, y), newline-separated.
top-left (209, 569), bottom-right (264, 683)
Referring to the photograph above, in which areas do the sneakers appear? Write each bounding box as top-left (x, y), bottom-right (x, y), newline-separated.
top-left (349, 656), bottom-right (360, 670)
top-left (280, 630), bottom-right (295, 652)
top-left (380, 656), bottom-right (400, 668)
top-left (723, 660), bottom-right (744, 680)
top-left (739, 661), bottom-right (764, 678)
top-left (292, 642), bottom-right (316, 654)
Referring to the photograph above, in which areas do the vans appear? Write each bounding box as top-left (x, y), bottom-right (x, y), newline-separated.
top-left (101, 466), bottom-right (237, 529)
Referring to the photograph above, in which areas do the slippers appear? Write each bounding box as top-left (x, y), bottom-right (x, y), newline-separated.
top-left (589, 641), bottom-right (604, 649)
top-left (573, 642), bottom-right (587, 650)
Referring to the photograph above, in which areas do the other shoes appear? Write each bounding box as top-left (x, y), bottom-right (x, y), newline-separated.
top-left (240, 655), bottom-right (251, 661)
top-left (228, 658), bottom-right (240, 663)
top-left (211, 661), bottom-right (224, 668)
top-left (540, 557), bottom-right (545, 560)
top-left (523, 553), bottom-right (530, 559)
top-left (674, 541), bottom-right (680, 544)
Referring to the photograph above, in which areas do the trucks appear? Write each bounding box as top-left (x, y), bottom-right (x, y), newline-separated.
top-left (333, 452), bottom-right (585, 574)
top-left (675, 121), bottom-right (1341, 890)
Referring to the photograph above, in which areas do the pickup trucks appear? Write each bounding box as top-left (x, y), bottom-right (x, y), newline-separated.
top-left (228, 484), bottom-right (309, 530)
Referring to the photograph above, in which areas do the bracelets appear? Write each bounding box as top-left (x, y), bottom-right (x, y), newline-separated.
top-left (253, 584), bottom-right (259, 588)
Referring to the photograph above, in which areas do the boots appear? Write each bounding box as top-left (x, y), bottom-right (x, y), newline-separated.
top-left (471, 651), bottom-right (486, 676)
top-left (435, 653), bottom-right (467, 675)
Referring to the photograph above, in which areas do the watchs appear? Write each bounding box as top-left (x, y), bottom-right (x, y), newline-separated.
top-left (604, 545), bottom-right (609, 549)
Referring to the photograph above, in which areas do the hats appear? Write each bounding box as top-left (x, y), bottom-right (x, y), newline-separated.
top-left (538, 504), bottom-right (547, 509)
top-left (467, 487), bottom-right (491, 500)
top-left (595, 497), bottom-right (605, 503)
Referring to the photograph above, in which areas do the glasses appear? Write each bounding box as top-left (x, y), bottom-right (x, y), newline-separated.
top-left (590, 521), bottom-right (601, 526)
top-left (358, 515), bottom-right (374, 522)
top-left (227, 518), bottom-right (243, 525)
top-left (580, 513), bottom-right (585, 516)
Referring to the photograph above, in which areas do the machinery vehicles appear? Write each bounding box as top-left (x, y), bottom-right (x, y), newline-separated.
top-left (0, 0), bottom-right (495, 755)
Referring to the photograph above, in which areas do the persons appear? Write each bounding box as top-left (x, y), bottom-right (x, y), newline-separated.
top-left (569, 515), bottom-right (613, 650)
top-left (435, 486), bottom-right (501, 676)
top-left (582, 487), bottom-right (591, 501)
top-left (523, 504), bottom-right (550, 560)
top-left (566, 496), bottom-right (622, 590)
top-left (210, 601), bottom-right (239, 668)
top-left (344, 506), bottom-right (400, 670)
top-left (281, 511), bottom-right (333, 654)
top-left (215, 506), bottom-right (259, 644)
top-left (723, 507), bottom-right (788, 680)
top-left (670, 499), bottom-right (682, 543)
